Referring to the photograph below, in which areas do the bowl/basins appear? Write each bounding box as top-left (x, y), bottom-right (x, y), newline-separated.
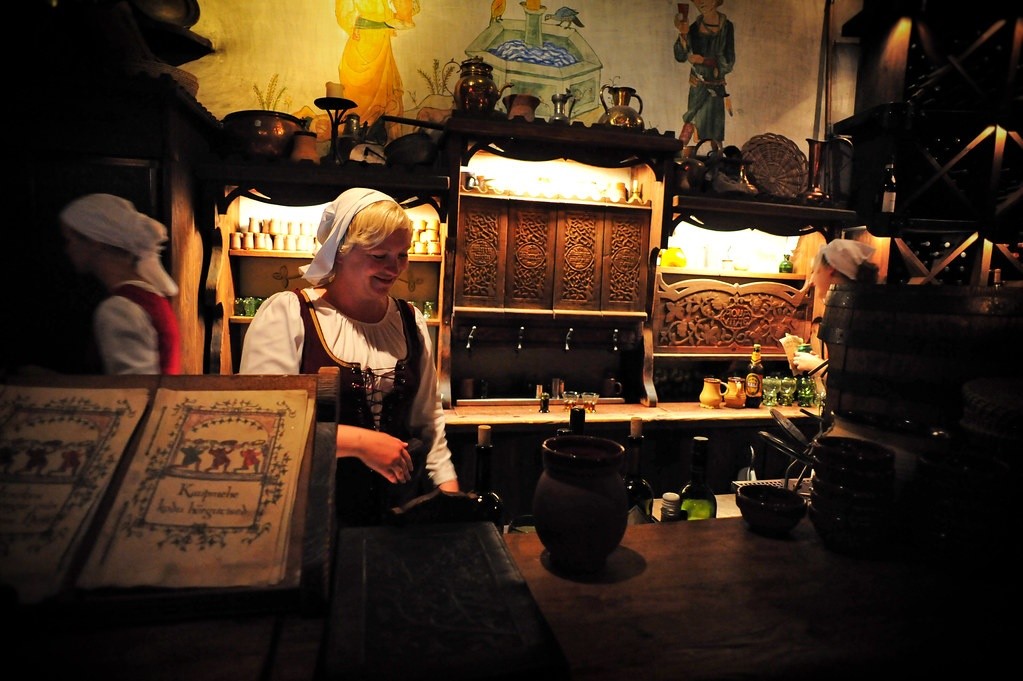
top-left (222, 110), bottom-right (301, 158)
top-left (734, 435), bottom-right (1006, 583)
top-left (383, 131), bottom-right (440, 168)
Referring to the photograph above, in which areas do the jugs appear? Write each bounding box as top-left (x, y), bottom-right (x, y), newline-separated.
top-left (674, 138), bottom-right (723, 193)
top-left (795, 137), bottom-right (854, 208)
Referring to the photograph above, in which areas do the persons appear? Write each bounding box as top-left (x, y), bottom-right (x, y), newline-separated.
top-left (242, 187), bottom-right (459, 527)
top-left (792, 239), bottom-right (875, 379)
top-left (59, 193), bottom-right (180, 375)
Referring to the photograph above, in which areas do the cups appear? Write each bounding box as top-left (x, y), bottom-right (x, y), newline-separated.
top-left (408, 217), bottom-right (441, 255)
top-left (581, 391), bottom-right (599, 412)
top-left (601, 376), bottom-right (623, 398)
top-left (230, 217), bottom-right (321, 251)
top-left (724, 376), bottom-right (746, 407)
top-left (232, 295), bottom-right (266, 319)
top-left (699, 376), bottom-right (729, 407)
top-left (408, 302), bottom-right (437, 320)
top-left (762, 374), bottom-right (815, 407)
top-left (561, 391), bottom-right (580, 412)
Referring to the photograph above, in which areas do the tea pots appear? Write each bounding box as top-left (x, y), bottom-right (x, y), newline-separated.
top-left (442, 54), bottom-right (514, 112)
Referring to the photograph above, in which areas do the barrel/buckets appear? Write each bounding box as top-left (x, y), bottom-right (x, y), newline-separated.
top-left (814, 279), bottom-right (1023, 440)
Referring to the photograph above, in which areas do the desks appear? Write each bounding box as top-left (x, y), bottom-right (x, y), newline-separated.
top-left (441, 399), bottom-right (825, 435)
top-left (505, 508), bottom-right (964, 681)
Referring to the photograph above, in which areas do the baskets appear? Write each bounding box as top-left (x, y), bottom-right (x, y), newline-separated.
top-left (741, 133), bottom-right (810, 203)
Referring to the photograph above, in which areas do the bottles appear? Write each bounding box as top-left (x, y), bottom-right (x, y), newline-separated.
top-left (598, 83), bottom-right (645, 128)
top-left (880, 153), bottom-right (897, 214)
top-left (626, 416), bottom-right (654, 526)
top-left (534, 435), bottom-right (629, 571)
top-left (779, 254), bottom-right (793, 273)
top-left (680, 436), bottom-right (718, 521)
top-left (895, 236), bottom-right (1004, 287)
top-left (900, 20), bottom-right (1023, 216)
top-left (289, 129), bottom-right (320, 164)
top-left (660, 492), bottom-right (680, 523)
top-left (744, 344), bottom-right (765, 408)
top-left (461, 424), bottom-right (503, 533)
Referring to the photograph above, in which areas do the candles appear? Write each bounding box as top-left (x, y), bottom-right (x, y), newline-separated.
top-left (326, 82), bottom-right (345, 98)
